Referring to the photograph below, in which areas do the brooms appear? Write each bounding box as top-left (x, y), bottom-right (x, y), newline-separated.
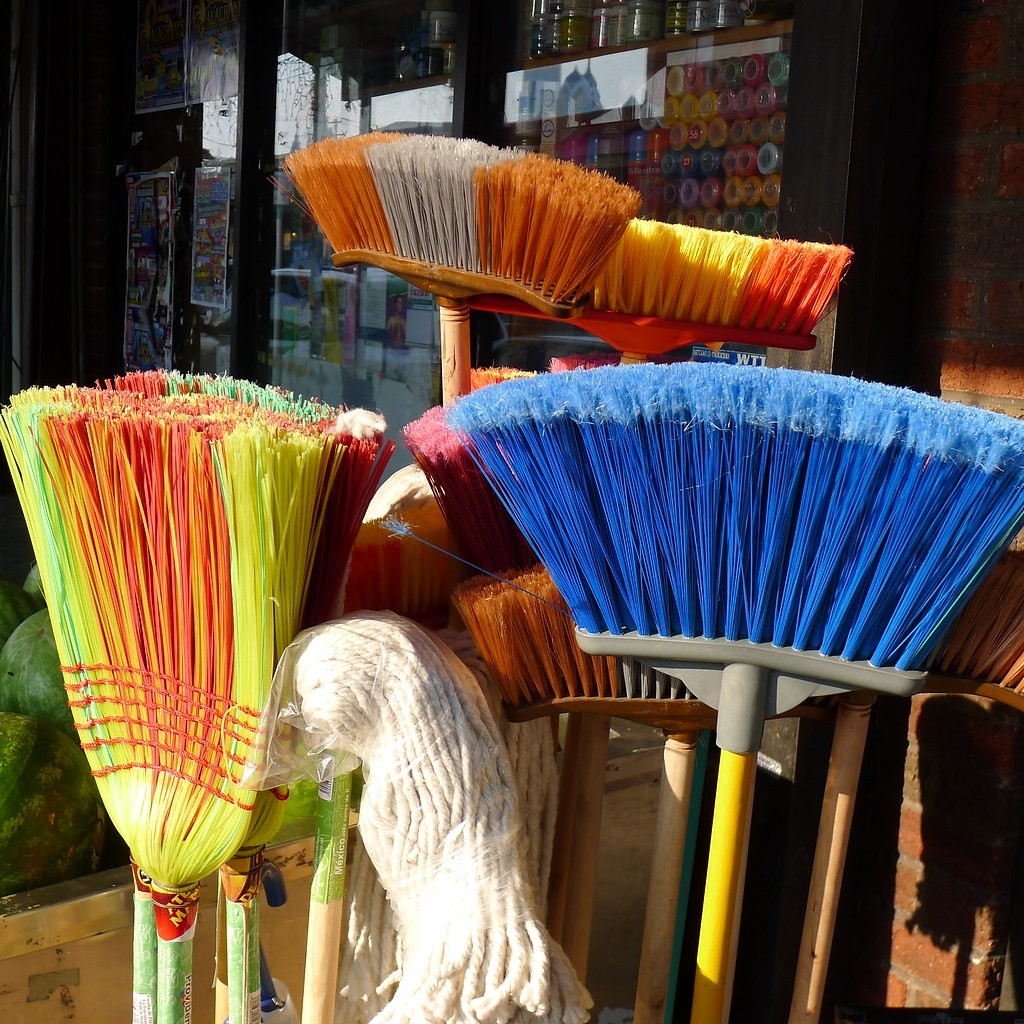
top-left (271, 128), bottom-right (1024, 1023)
top-left (0, 366), bottom-right (397, 1024)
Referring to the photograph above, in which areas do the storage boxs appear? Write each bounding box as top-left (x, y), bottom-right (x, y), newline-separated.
top-left (3, 704), bottom-right (693, 1024)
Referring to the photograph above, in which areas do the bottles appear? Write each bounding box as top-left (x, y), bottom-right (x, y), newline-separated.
top-left (544, 0), bottom-right (563, 58)
top-left (529, 0), bottom-right (547, 59)
top-left (559, 0), bottom-right (594, 54)
top-left (607, 0), bottom-right (628, 47)
top-left (588, 0), bottom-right (612, 51)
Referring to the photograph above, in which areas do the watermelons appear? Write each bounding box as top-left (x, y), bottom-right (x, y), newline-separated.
top-left (0, 559), bottom-right (108, 897)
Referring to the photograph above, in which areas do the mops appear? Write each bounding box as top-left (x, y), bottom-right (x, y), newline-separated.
top-left (233, 605), bottom-right (595, 1024)
